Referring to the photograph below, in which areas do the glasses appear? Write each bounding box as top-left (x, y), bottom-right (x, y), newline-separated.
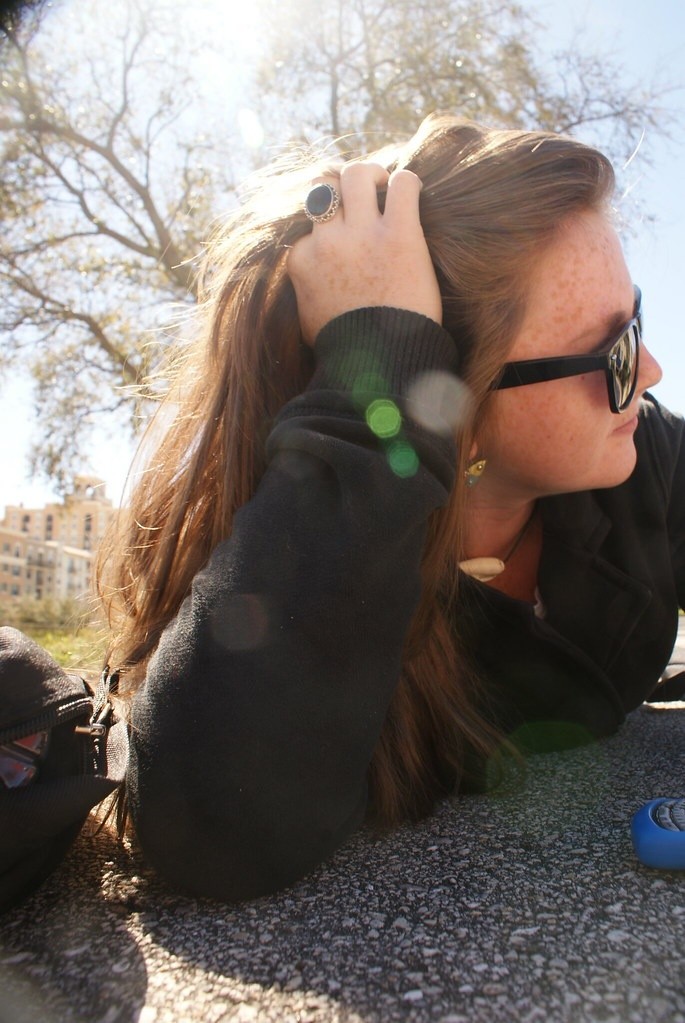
top-left (486, 284), bottom-right (643, 414)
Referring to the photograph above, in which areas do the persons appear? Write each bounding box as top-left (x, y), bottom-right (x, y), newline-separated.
top-left (104, 112), bottom-right (685, 902)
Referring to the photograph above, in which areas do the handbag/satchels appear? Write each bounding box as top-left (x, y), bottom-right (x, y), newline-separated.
top-left (0, 628), bottom-right (129, 911)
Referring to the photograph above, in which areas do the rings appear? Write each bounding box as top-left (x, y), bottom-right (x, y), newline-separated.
top-left (304, 183), bottom-right (342, 225)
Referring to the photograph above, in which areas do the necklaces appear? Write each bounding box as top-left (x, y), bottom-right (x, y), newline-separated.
top-left (457, 497), bottom-right (538, 582)
